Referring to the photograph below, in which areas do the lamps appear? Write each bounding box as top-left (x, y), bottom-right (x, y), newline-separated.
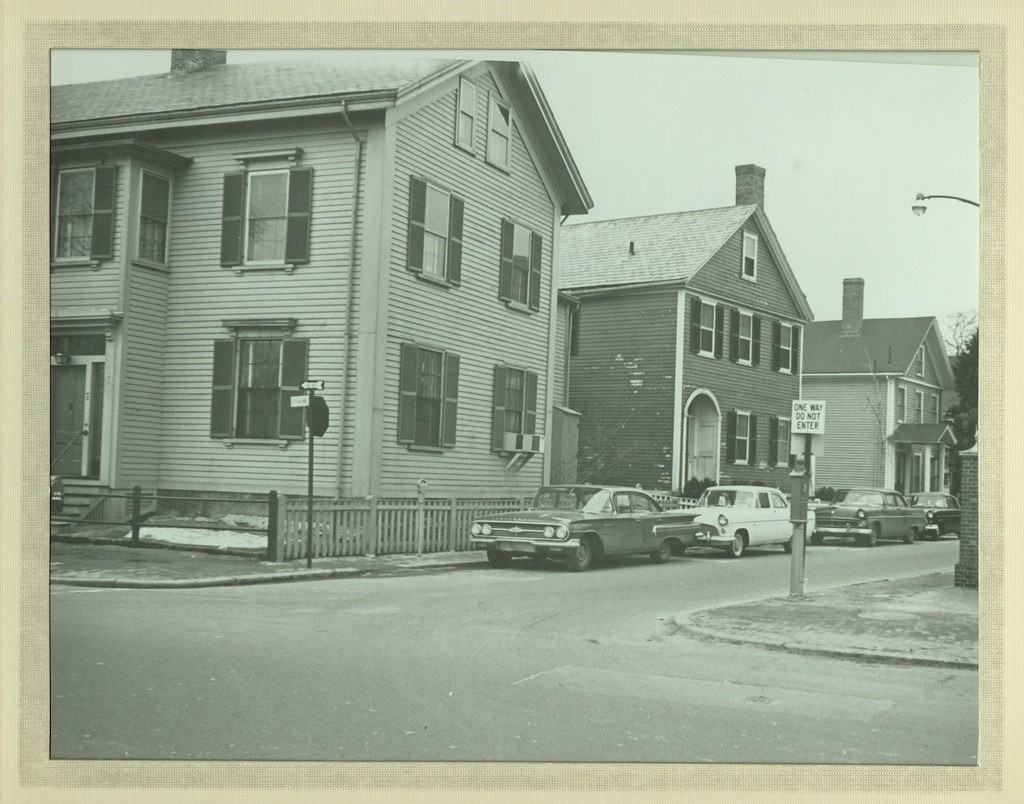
top-left (54, 348), bottom-right (71, 364)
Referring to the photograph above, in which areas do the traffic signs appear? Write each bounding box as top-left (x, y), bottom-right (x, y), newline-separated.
top-left (299, 380), bottom-right (324, 390)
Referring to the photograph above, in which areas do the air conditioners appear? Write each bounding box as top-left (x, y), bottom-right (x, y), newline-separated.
top-left (503, 433), bottom-right (547, 454)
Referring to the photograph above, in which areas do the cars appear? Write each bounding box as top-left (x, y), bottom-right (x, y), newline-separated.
top-left (469, 484), bottom-right (702, 572)
top-left (812, 488), bottom-right (926, 544)
top-left (686, 485), bottom-right (816, 556)
top-left (904, 492), bottom-right (961, 541)
